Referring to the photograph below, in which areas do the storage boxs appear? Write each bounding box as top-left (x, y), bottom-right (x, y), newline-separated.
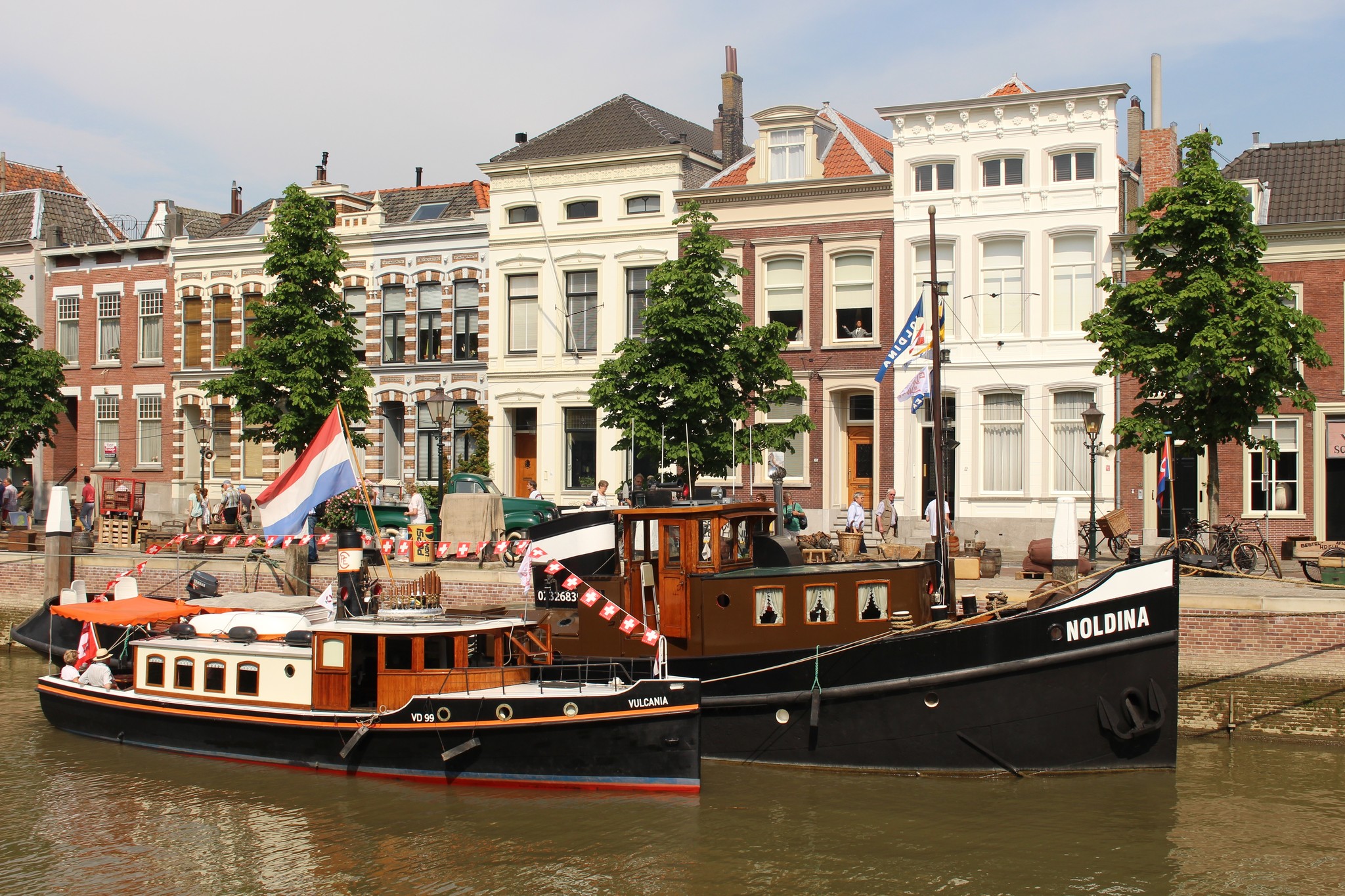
top-left (1096, 508), bottom-right (1131, 538)
top-left (363, 547), bottom-right (385, 566)
top-left (8, 529), bottom-right (39, 552)
top-left (140, 531), bottom-right (179, 553)
top-left (443, 541), bottom-right (501, 562)
top-left (104, 490), bottom-right (151, 530)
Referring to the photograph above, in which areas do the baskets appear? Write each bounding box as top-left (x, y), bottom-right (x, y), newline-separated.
top-left (836, 530), bottom-right (865, 557)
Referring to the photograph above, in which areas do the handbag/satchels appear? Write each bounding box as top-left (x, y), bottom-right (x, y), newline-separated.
top-left (241, 505), bottom-right (248, 515)
top-left (799, 515), bottom-right (807, 530)
top-left (423, 503), bottom-right (433, 521)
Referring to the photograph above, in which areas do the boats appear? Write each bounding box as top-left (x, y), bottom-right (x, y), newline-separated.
top-left (34, 398), bottom-right (702, 797)
top-left (12, 205), bottom-right (1181, 783)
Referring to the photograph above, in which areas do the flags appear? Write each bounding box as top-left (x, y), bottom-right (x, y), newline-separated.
top-left (1155, 436), bottom-right (1171, 515)
top-left (74, 531), bottom-right (333, 674)
top-left (361, 531), bottom-right (660, 678)
top-left (255, 404), bottom-right (359, 551)
top-left (873, 292), bottom-right (945, 414)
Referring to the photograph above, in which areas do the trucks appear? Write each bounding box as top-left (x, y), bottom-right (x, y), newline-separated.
top-left (354, 473), bottom-right (561, 563)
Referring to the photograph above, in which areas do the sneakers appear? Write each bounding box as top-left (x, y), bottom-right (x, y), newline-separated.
top-left (86, 528), bottom-right (92, 532)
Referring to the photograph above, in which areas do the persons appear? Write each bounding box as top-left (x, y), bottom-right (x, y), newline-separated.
top-left (874, 487), bottom-right (898, 544)
top-left (756, 493), bottom-right (766, 502)
top-left (924, 489), bottom-right (955, 542)
top-left (1, 477), bottom-right (18, 521)
top-left (618, 473), bottom-right (644, 507)
top-left (586, 480), bottom-right (611, 507)
top-left (403, 482), bottom-right (426, 524)
top-left (236, 485), bottom-right (253, 534)
top-left (17, 477), bottom-right (34, 530)
top-left (845, 492), bottom-right (867, 554)
top-left (0, 477), bottom-right (5, 517)
top-left (841, 321), bottom-right (872, 338)
top-left (782, 491), bottom-right (805, 544)
top-left (526, 480), bottom-right (542, 500)
top-left (60, 649), bottom-right (81, 683)
top-left (186, 479), bottom-right (239, 533)
top-left (113, 480), bottom-right (130, 515)
top-left (77, 648), bottom-right (120, 691)
top-left (79, 475), bottom-right (95, 532)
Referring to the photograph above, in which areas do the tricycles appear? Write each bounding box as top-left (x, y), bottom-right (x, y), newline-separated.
top-left (1279, 540), bottom-right (1345, 583)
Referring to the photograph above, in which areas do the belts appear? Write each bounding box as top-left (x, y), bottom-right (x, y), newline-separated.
top-left (889, 525), bottom-right (895, 528)
top-left (84, 502), bottom-right (94, 503)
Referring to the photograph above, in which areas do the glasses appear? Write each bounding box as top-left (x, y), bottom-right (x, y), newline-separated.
top-left (889, 494), bottom-right (896, 496)
top-left (756, 498), bottom-right (761, 500)
top-left (859, 497), bottom-right (864, 499)
top-left (635, 479), bottom-right (643, 483)
top-left (602, 486), bottom-right (608, 488)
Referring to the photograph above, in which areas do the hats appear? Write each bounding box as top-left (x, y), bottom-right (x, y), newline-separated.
top-left (221, 480), bottom-right (231, 485)
top-left (21, 478), bottom-right (30, 481)
top-left (92, 648), bottom-right (114, 661)
top-left (236, 485), bottom-right (246, 490)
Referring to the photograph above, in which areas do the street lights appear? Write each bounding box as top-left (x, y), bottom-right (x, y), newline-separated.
top-left (426, 384), bottom-right (457, 561)
top-left (1080, 399), bottom-right (1105, 561)
top-left (193, 420), bottom-right (214, 489)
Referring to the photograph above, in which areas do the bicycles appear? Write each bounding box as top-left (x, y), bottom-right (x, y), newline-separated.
top-left (1076, 509), bottom-right (1134, 560)
top-left (1152, 513), bottom-right (1284, 581)
